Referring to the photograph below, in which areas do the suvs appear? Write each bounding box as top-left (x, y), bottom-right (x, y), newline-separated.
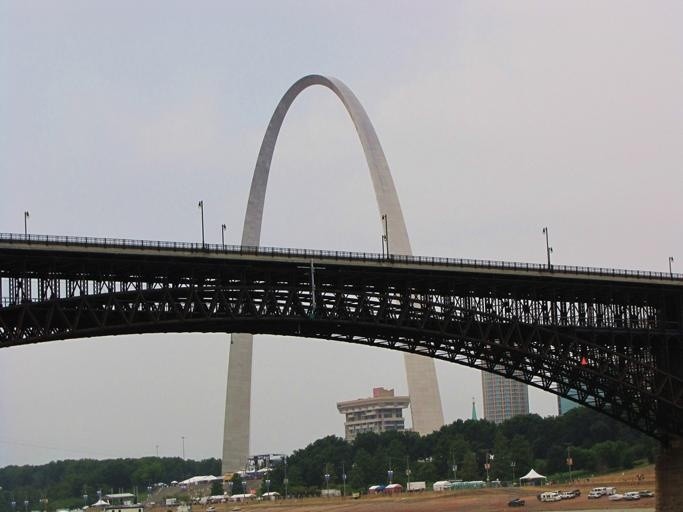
top-left (537, 486), bottom-right (653, 502)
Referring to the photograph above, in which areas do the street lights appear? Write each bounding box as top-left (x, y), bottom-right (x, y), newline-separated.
top-left (22, 211), bottom-right (30, 237)
top-left (542, 227), bottom-right (552, 270)
top-left (667, 255), bottom-right (673, 279)
top-left (379, 213), bottom-right (388, 260)
top-left (198, 201), bottom-right (203, 249)
top-left (221, 224), bottom-right (226, 250)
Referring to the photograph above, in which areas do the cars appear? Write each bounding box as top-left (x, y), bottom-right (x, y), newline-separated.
top-left (509, 499), bottom-right (524, 507)
